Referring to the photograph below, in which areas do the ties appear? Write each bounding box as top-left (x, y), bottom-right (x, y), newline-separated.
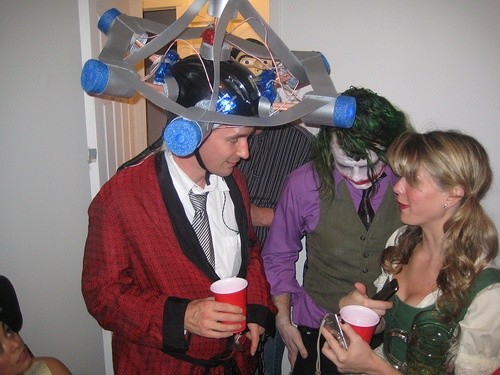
top-left (358, 189), bottom-right (376, 228)
top-left (188, 187), bottom-right (216, 271)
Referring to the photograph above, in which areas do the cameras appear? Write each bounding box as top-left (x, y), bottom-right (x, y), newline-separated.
top-left (325, 314), bottom-right (349, 351)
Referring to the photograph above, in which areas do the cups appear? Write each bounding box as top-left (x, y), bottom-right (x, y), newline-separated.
top-left (209, 277), bottom-right (248, 334)
top-left (340, 305), bottom-right (380, 345)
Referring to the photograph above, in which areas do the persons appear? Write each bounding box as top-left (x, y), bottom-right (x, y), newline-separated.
top-left (0, 320), bottom-right (73, 375)
top-left (322, 130), bottom-right (500, 375)
top-left (80, 54), bottom-right (276, 375)
top-left (232, 39), bottom-right (321, 375)
top-left (261, 88), bottom-right (416, 375)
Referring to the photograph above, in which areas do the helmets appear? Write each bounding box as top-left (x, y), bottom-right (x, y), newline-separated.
top-left (164, 52), bottom-right (261, 128)
